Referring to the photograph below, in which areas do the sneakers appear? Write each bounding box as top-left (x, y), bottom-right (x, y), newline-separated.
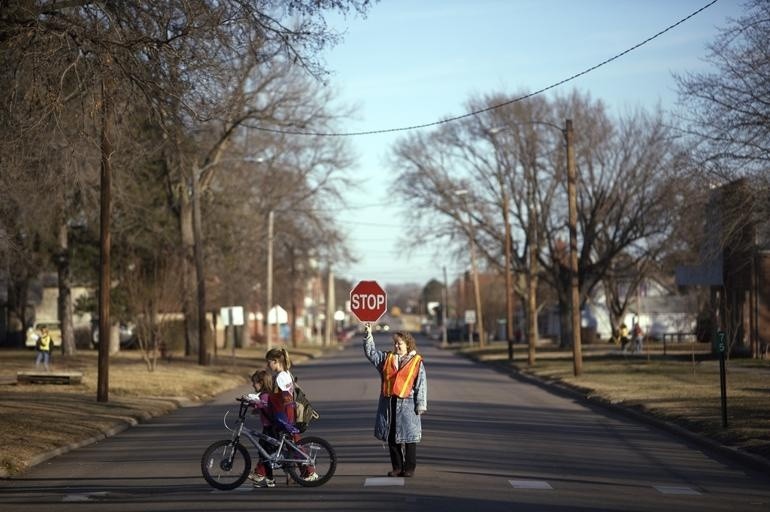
top-left (252, 476), bottom-right (275, 488)
top-left (247, 471), bottom-right (264, 482)
top-left (294, 472), bottom-right (319, 486)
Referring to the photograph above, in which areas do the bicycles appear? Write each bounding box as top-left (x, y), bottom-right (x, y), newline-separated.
top-left (200, 393), bottom-right (337, 491)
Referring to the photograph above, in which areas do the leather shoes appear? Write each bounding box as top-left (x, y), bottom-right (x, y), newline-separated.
top-left (401, 466), bottom-right (413, 477)
top-left (388, 469), bottom-right (402, 477)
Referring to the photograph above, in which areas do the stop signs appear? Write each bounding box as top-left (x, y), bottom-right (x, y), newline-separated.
top-left (349, 280), bottom-right (388, 325)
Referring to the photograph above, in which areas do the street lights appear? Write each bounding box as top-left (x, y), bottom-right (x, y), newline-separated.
top-left (191, 155), bottom-right (265, 364)
top-left (485, 116), bottom-right (585, 376)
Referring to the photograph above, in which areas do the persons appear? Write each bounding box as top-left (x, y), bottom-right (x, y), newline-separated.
top-left (633, 324), bottom-right (643, 352)
top-left (364, 324), bottom-right (427, 478)
top-left (241, 370), bottom-right (315, 488)
top-left (35, 326), bottom-right (51, 371)
top-left (248, 348), bottom-right (318, 483)
top-left (620, 325), bottom-right (631, 352)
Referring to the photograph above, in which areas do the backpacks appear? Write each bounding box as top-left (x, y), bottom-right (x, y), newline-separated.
top-left (284, 370), bottom-right (319, 424)
top-left (260, 391), bottom-right (297, 432)
